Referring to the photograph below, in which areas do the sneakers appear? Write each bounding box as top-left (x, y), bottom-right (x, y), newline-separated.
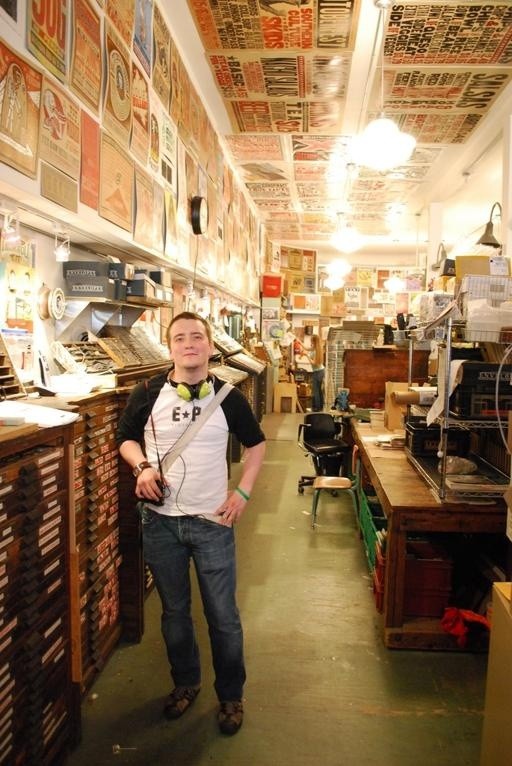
top-left (217, 701), bottom-right (244, 734)
top-left (164, 687), bottom-right (200, 721)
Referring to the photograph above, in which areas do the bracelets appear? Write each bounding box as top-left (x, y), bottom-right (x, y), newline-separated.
top-left (235, 486), bottom-right (250, 502)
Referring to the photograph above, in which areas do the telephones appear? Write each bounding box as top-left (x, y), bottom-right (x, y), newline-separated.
top-left (32, 349), bottom-right (57, 395)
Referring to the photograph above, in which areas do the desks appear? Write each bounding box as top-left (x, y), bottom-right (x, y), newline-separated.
top-left (350, 411), bottom-right (508, 652)
top-left (479, 581), bottom-right (512, 766)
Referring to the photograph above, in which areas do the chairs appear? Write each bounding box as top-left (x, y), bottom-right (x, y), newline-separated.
top-left (297, 413), bottom-right (348, 497)
top-left (311, 444), bottom-right (361, 529)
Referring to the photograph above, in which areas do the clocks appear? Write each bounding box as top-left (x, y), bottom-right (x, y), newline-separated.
top-left (191, 196), bottom-right (208, 234)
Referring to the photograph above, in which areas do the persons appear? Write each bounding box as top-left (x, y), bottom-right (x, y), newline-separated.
top-left (115, 310), bottom-right (268, 736)
top-left (296, 334), bottom-right (326, 413)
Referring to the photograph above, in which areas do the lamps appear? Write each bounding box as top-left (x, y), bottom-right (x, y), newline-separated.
top-left (52, 231), bottom-right (71, 263)
top-left (352, 0), bottom-right (416, 170)
top-left (475, 202), bottom-right (502, 255)
top-left (432, 243), bottom-right (447, 271)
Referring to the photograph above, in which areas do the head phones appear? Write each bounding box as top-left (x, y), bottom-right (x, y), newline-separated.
top-left (166, 368), bottom-right (216, 403)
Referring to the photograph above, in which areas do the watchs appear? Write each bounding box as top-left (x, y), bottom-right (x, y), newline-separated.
top-left (132, 460), bottom-right (152, 479)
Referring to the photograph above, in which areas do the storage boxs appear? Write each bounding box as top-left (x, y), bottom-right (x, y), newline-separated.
top-left (447, 255), bottom-right (512, 294)
top-left (357, 487), bottom-right (452, 619)
